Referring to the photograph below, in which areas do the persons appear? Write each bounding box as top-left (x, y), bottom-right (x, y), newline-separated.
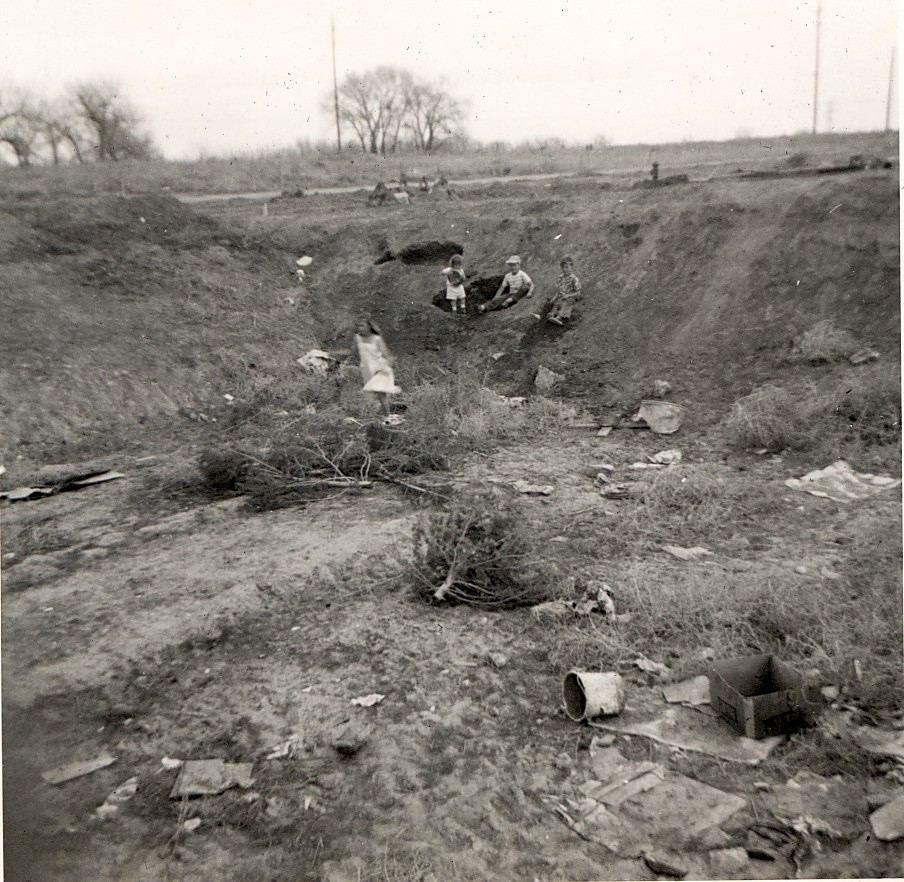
top-left (440, 254), bottom-right (468, 315)
top-left (351, 311), bottom-right (403, 426)
top-left (473, 254), bottom-right (535, 315)
top-left (531, 258), bottom-right (582, 326)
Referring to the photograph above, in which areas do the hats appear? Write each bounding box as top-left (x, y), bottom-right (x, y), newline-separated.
top-left (559, 255), bottom-right (573, 264)
top-left (505, 255), bottom-right (521, 265)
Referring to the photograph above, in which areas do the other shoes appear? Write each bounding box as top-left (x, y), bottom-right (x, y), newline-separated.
top-left (546, 313), bottom-right (554, 321)
top-left (501, 300), bottom-right (508, 308)
top-left (549, 317), bottom-right (562, 325)
top-left (474, 304), bottom-right (482, 316)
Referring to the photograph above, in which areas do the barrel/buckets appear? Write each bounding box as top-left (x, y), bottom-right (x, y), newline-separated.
top-left (562, 671), bottom-right (624, 722)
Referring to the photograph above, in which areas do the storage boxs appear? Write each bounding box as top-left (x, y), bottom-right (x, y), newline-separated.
top-left (708, 653), bottom-right (804, 742)
